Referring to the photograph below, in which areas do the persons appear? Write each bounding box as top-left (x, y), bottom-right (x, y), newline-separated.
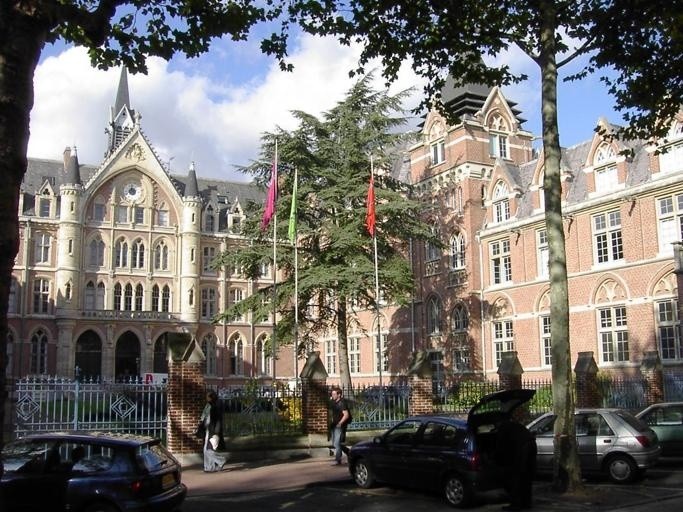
top-left (197, 390), bottom-right (225, 452)
top-left (329, 388), bottom-right (350, 466)
top-left (487, 408), bottom-right (538, 512)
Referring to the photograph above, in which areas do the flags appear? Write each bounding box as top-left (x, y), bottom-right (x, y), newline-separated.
top-left (365, 174), bottom-right (377, 237)
top-left (261, 165), bottom-right (275, 231)
top-left (287, 179), bottom-right (301, 244)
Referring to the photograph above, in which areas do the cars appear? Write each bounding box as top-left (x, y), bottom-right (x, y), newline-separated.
top-left (527, 409), bottom-right (660, 486)
top-left (0, 430), bottom-right (186, 511)
top-left (637, 403), bottom-right (683, 442)
top-left (347, 389), bottom-right (538, 507)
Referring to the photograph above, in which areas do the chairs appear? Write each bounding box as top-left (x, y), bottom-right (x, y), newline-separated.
top-left (56, 445), bottom-right (84, 476)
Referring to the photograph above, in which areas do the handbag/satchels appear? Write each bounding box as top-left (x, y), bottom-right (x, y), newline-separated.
top-left (193, 424), bottom-right (206, 441)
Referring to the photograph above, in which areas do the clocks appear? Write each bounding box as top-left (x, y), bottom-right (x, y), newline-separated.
top-left (118, 177), bottom-right (146, 205)
